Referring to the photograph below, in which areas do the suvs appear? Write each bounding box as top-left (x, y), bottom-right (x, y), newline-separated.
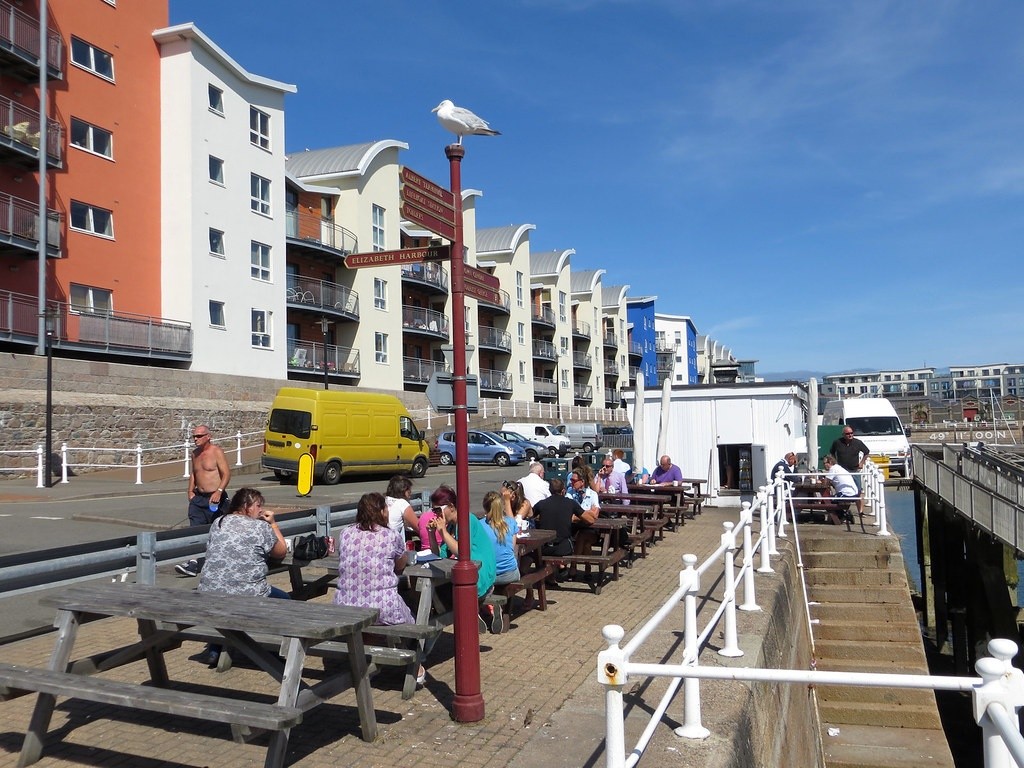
top-left (487, 430), bottom-right (548, 461)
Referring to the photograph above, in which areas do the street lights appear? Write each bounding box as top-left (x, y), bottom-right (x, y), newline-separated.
top-left (554, 354), bottom-right (560, 417)
top-left (610, 366), bottom-right (614, 420)
top-left (321, 316), bottom-right (329, 390)
top-left (43, 303), bottom-right (56, 487)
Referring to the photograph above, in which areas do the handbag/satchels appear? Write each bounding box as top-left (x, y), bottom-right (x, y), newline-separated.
top-left (292, 534), bottom-right (328, 559)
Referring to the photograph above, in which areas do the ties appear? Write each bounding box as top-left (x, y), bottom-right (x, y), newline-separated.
top-left (605, 474), bottom-right (610, 493)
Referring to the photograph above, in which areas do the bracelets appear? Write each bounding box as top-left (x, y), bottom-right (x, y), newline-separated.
top-left (217, 488), bottom-right (223, 492)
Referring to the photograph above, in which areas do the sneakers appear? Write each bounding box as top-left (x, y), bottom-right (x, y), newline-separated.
top-left (176, 560), bottom-right (197, 577)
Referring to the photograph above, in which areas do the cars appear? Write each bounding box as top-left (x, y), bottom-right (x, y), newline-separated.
top-left (602, 427), bottom-right (632, 434)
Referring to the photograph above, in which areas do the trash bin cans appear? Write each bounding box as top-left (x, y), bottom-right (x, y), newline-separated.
top-left (540, 457), bottom-right (568, 481)
top-left (622, 451), bottom-right (633, 468)
top-left (581, 452), bottom-right (606, 475)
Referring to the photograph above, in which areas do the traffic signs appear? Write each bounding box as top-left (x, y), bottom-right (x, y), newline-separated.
top-left (398, 165), bottom-right (455, 241)
top-left (343, 245), bottom-right (452, 270)
top-left (463, 263), bottom-right (501, 306)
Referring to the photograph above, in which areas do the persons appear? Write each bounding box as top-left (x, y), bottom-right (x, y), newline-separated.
top-left (516, 463), bottom-right (552, 507)
top-left (594, 458), bottom-right (630, 543)
top-left (820, 454), bottom-right (859, 524)
top-left (564, 465), bottom-right (601, 579)
top-left (566, 456), bottom-right (591, 487)
top-left (530, 478), bottom-right (595, 590)
top-left (612, 449), bottom-right (630, 484)
top-left (384, 475), bottom-right (419, 551)
top-left (478, 491), bottom-right (520, 586)
top-left (771, 452), bottom-right (802, 503)
top-left (418, 482), bottom-right (503, 634)
top-left (499, 480), bottom-right (536, 609)
top-left (830, 427), bottom-right (870, 518)
top-left (174, 425), bottom-right (232, 577)
top-left (649, 455), bottom-right (684, 507)
top-left (197, 488), bottom-right (295, 601)
top-left (333, 492), bottom-right (427, 685)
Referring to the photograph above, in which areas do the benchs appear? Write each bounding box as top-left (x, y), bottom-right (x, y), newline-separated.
top-left (0, 494), bottom-right (708, 768)
top-left (786, 504), bottom-right (842, 523)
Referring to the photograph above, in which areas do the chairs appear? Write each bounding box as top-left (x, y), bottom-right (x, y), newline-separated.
top-left (286, 287), bottom-right (353, 311)
top-left (4, 121), bottom-right (41, 149)
top-left (290, 348), bottom-right (309, 368)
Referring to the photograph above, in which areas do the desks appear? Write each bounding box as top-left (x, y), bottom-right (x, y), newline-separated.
top-left (574, 519), bottom-right (627, 530)
top-left (598, 494), bottom-right (671, 500)
top-left (682, 479), bottom-right (707, 487)
top-left (516, 530), bottom-right (556, 546)
top-left (38, 585), bottom-right (378, 767)
top-left (272, 549), bottom-right (483, 624)
top-left (627, 486), bottom-right (691, 494)
top-left (601, 504), bottom-right (653, 514)
top-left (793, 476), bottom-right (832, 504)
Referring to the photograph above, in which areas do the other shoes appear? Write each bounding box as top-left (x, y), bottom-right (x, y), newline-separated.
top-left (422, 628), bottom-right (443, 655)
top-left (477, 615), bottom-right (488, 634)
top-left (417, 665), bottom-right (425, 683)
top-left (859, 512), bottom-right (866, 518)
top-left (491, 603), bottom-right (503, 633)
top-left (520, 596), bottom-right (534, 610)
top-left (568, 569), bottom-right (577, 581)
top-left (583, 574), bottom-right (593, 584)
top-left (846, 510), bottom-right (855, 525)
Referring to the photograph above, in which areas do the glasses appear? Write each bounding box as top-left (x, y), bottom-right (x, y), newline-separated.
top-left (602, 464), bottom-right (614, 468)
top-left (502, 479), bottom-right (516, 496)
top-left (432, 503), bottom-right (449, 515)
top-left (788, 452), bottom-right (794, 460)
top-left (844, 432), bottom-right (856, 435)
top-left (193, 433), bottom-right (209, 438)
top-left (569, 477), bottom-right (582, 483)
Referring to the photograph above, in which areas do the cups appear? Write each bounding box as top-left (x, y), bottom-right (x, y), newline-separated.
top-left (810, 478), bottom-right (817, 485)
top-left (802, 476), bottom-right (805, 482)
top-left (285, 538), bottom-right (292, 553)
top-left (608, 486), bottom-right (615, 494)
top-left (672, 481), bottom-right (678, 486)
top-left (408, 551), bottom-right (417, 566)
top-left (521, 520), bottom-right (529, 530)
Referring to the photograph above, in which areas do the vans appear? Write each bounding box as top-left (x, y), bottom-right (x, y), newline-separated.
top-left (551, 422), bottom-right (604, 452)
top-left (822, 398), bottom-right (913, 477)
top-left (502, 422), bottom-right (571, 458)
top-left (435, 429), bottom-right (527, 467)
top-left (261, 386), bottom-right (430, 485)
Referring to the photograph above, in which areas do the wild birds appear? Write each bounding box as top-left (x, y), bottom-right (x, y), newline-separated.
top-left (431, 100), bottom-right (502, 145)
